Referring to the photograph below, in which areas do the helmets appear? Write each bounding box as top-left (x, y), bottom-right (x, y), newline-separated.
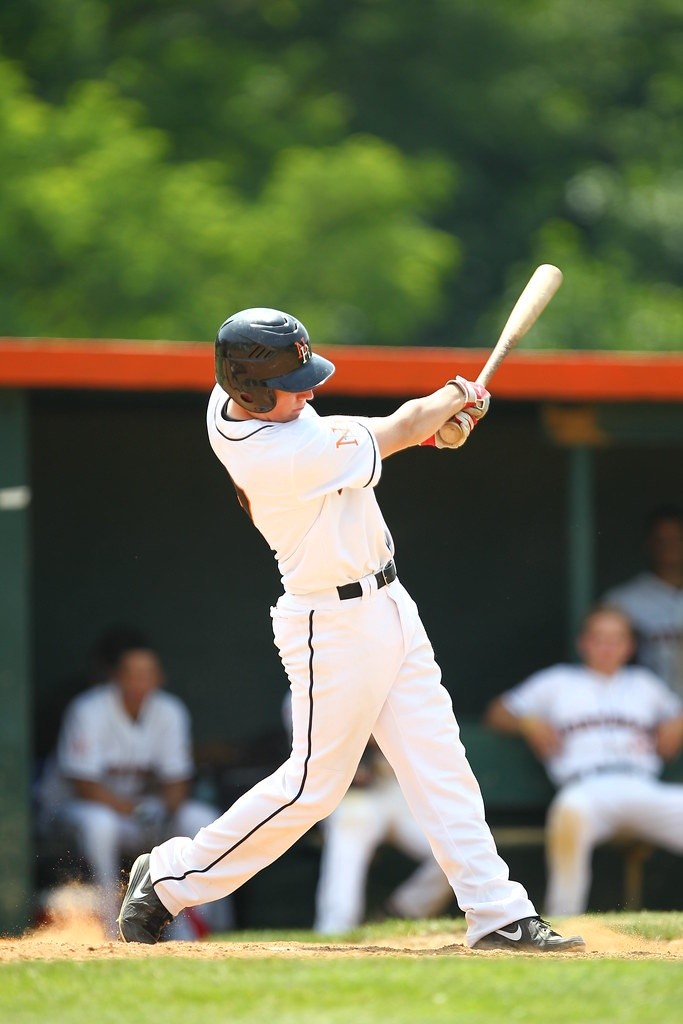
top-left (213, 308), bottom-right (335, 414)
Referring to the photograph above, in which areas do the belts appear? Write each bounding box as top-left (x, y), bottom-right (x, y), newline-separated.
top-left (336, 560), bottom-right (398, 600)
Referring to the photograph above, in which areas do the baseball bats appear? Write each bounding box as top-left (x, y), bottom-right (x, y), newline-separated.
top-left (436, 259), bottom-right (568, 449)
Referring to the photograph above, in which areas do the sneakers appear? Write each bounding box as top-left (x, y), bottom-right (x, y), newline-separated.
top-left (473, 916), bottom-right (586, 954)
top-left (116, 853), bottom-right (175, 945)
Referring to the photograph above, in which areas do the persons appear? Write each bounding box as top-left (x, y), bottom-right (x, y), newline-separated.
top-left (487, 607), bottom-right (683, 915)
top-left (38, 648), bottom-right (235, 940)
top-left (594, 514), bottom-right (683, 912)
top-left (280, 676), bottom-right (455, 936)
top-left (117, 306), bottom-right (587, 955)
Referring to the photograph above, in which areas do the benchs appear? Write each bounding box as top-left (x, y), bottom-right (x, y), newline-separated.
top-left (269, 716), bottom-right (655, 918)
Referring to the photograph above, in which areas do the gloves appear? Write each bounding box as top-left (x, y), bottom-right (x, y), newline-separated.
top-left (446, 375), bottom-right (491, 420)
top-left (419, 411), bottom-right (479, 450)
